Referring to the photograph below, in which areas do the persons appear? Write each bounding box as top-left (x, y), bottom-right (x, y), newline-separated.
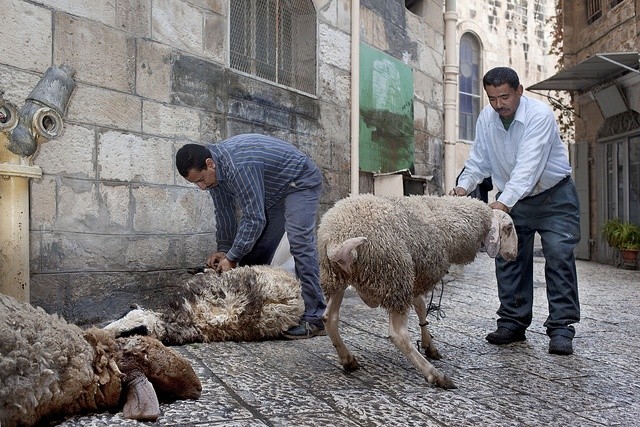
top-left (448, 67), bottom-right (581, 356)
top-left (176, 133), bottom-right (329, 339)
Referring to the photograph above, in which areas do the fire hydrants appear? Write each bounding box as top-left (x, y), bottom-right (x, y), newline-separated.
top-left (0, 64), bottom-right (77, 303)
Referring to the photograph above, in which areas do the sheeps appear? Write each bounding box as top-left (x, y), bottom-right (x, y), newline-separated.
top-left (0, 295), bottom-right (201, 426)
top-left (100, 264), bottom-right (305, 344)
top-left (317, 193), bottom-right (518, 390)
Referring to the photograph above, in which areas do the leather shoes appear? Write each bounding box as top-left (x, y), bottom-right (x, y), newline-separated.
top-left (548, 326), bottom-right (574, 354)
top-left (484, 325), bottom-right (526, 345)
top-left (281, 319), bottom-right (326, 340)
